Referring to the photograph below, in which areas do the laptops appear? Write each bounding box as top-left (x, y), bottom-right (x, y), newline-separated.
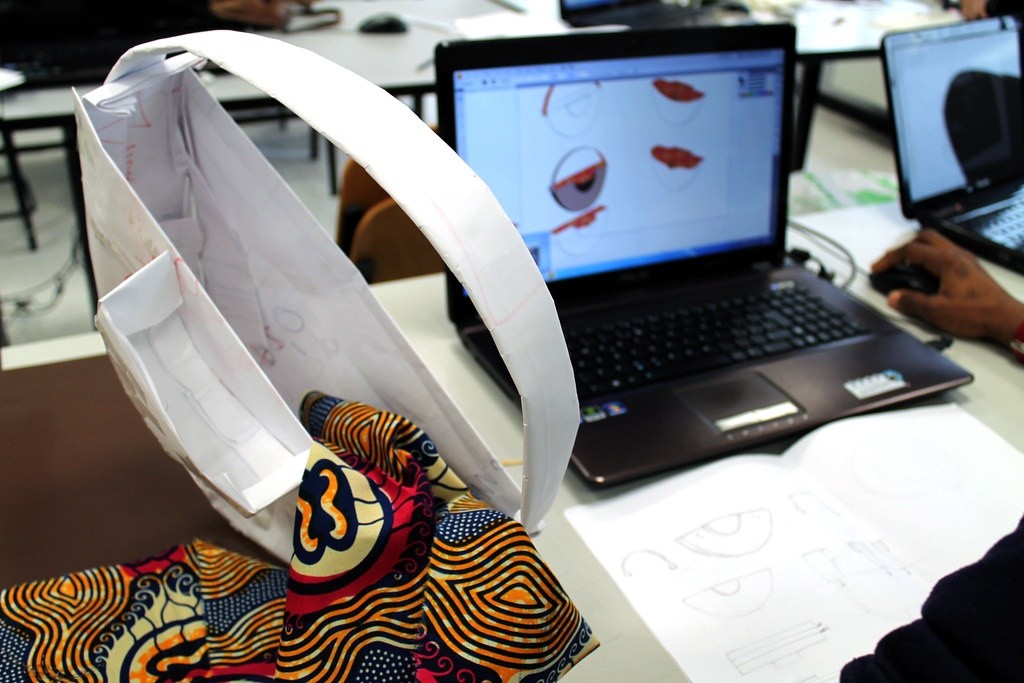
top-left (430, 0), bottom-right (973, 490)
top-left (883, 15), bottom-right (1024, 275)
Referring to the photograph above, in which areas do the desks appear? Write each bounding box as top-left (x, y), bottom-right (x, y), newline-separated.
top-left (0, 0), bottom-right (1024, 683)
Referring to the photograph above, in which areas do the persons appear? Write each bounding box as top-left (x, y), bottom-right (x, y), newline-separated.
top-left (838, 227), bottom-right (1024, 682)
top-left (0, 0), bottom-right (289, 66)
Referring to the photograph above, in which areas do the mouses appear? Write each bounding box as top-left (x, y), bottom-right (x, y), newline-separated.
top-left (361, 15), bottom-right (406, 34)
top-left (867, 267), bottom-right (942, 296)
